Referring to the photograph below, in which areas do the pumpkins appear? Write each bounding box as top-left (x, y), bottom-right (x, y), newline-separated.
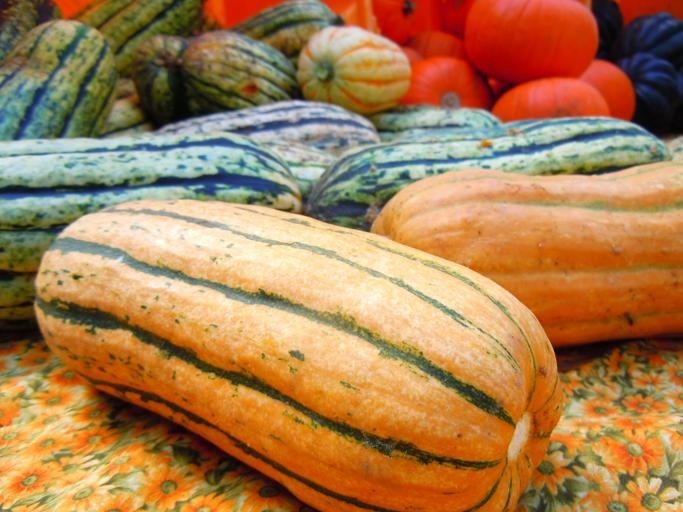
top-left (0, 0), bottom-right (683, 511)
